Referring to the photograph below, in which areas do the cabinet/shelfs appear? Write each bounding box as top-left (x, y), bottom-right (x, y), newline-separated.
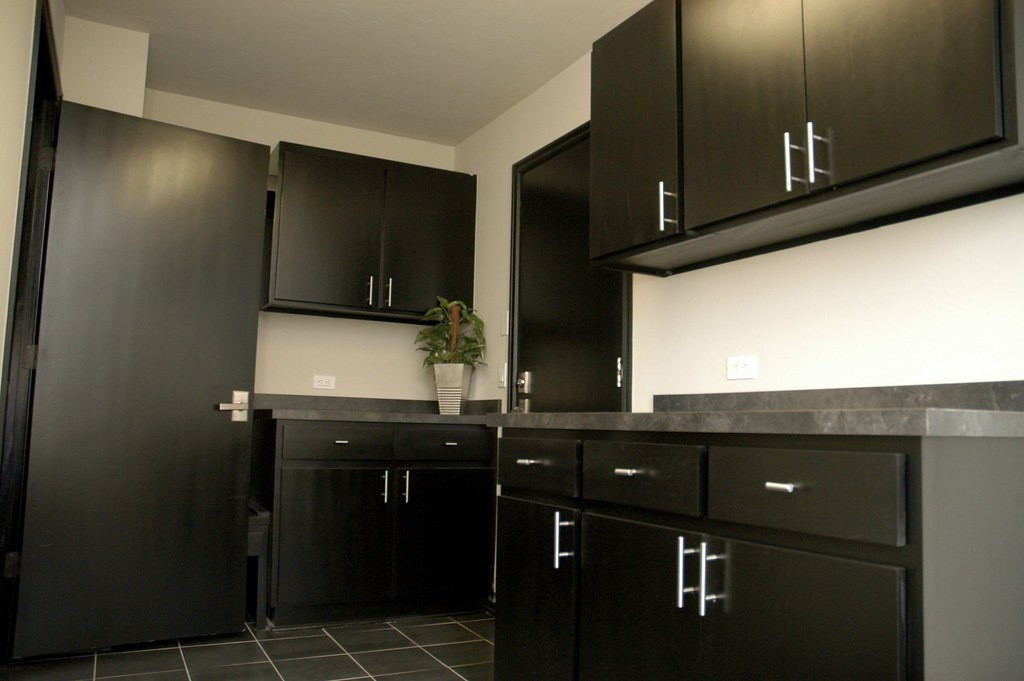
top-left (253, 408), bottom-right (497, 628)
top-left (588, 0), bottom-right (680, 276)
top-left (680, 0), bottom-right (1023, 274)
top-left (260, 139), bottom-right (477, 326)
top-left (494, 426), bottom-right (584, 680)
top-left (577, 429), bottom-right (1023, 681)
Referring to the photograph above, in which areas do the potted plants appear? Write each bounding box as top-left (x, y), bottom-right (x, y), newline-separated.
top-left (412, 296), bottom-right (486, 415)
